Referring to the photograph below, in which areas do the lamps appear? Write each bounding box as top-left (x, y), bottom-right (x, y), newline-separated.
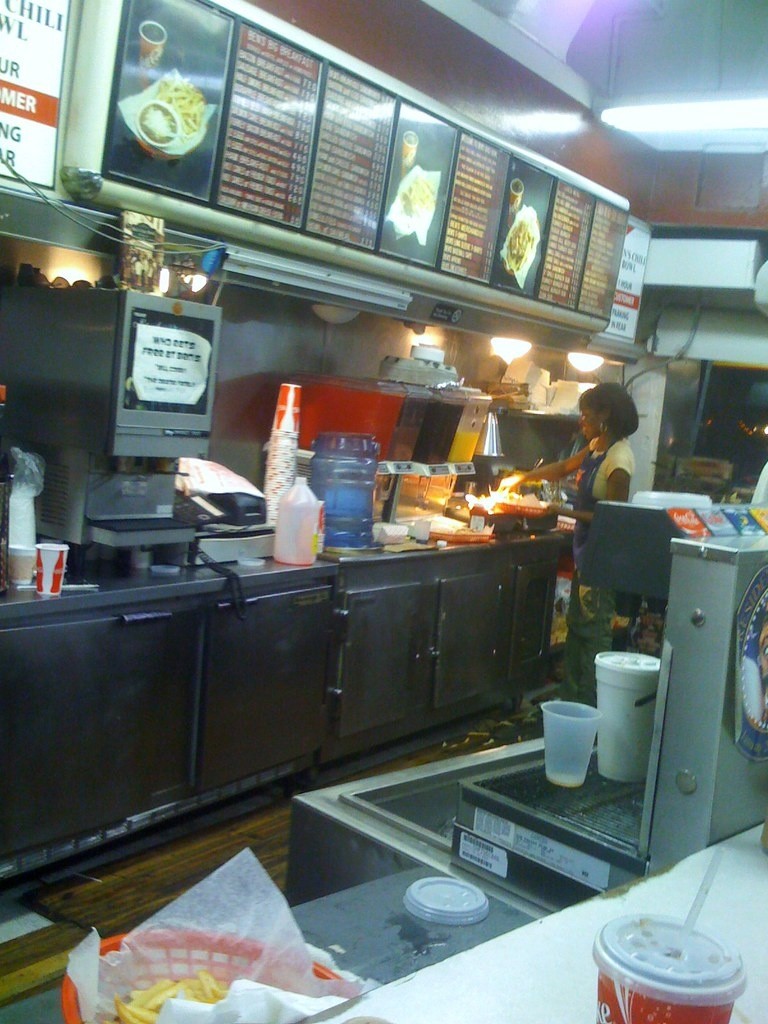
top-left (668, 360), bottom-right (701, 376)
top-left (567, 352), bottom-right (604, 373)
top-left (594, 84), bottom-right (768, 132)
top-left (491, 336), bottom-right (532, 358)
top-left (311, 304), bottom-right (359, 325)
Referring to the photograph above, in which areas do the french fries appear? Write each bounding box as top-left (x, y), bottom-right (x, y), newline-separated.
top-left (153, 76), bottom-right (205, 136)
top-left (507, 219), bottom-right (533, 271)
top-left (407, 175), bottom-right (435, 210)
top-left (112, 971), bottom-right (230, 1024)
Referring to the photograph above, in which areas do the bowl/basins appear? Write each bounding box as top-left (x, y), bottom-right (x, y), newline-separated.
top-left (138, 99), bottom-right (182, 146)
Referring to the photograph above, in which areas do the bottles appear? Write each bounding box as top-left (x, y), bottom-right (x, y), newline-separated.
top-left (311, 434), bottom-right (379, 548)
top-left (470, 504), bottom-right (485, 532)
top-left (272, 475), bottom-right (320, 567)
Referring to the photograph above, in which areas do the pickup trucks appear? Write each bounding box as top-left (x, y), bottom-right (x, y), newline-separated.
top-left (660, 436), bottom-right (732, 490)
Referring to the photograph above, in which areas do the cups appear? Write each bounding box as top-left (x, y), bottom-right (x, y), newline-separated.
top-left (263, 384), bottom-right (326, 555)
top-left (541, 700), bottom-right (603, 787)
top-left (415, 521), bottom-right (431, 544)
top-left (34, 543), bottom-right (69, 598)
top-left (508, 178), bottom-right (524, 229)
top-left (138, 19), bottom-right (166, 86)
top-left (594, 650), bottom-right (661, 781)
top-left (401, 131), bottom-right (419, 177)
top-left (593, 913), bottom-right (748, 1024)
top-left (9, 544), bottom-right (37, 585)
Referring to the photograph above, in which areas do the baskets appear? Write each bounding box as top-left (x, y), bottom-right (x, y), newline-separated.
top-left (495, 501), bottom-right (545, 520)
top-left (429, 531), bottom-right (492, 542)
top-left (59, 928), bottom-right (363, 1024)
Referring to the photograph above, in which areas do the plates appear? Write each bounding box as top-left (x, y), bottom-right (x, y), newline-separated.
top-left (504, 219), bottom-right (540, 274)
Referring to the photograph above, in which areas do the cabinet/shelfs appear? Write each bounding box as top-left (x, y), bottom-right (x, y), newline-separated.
top-left (1, 539), bottom-right (562, 882)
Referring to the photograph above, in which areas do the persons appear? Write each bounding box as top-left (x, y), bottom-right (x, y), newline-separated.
top-left (498, 382), bottom-right (639, 706)
